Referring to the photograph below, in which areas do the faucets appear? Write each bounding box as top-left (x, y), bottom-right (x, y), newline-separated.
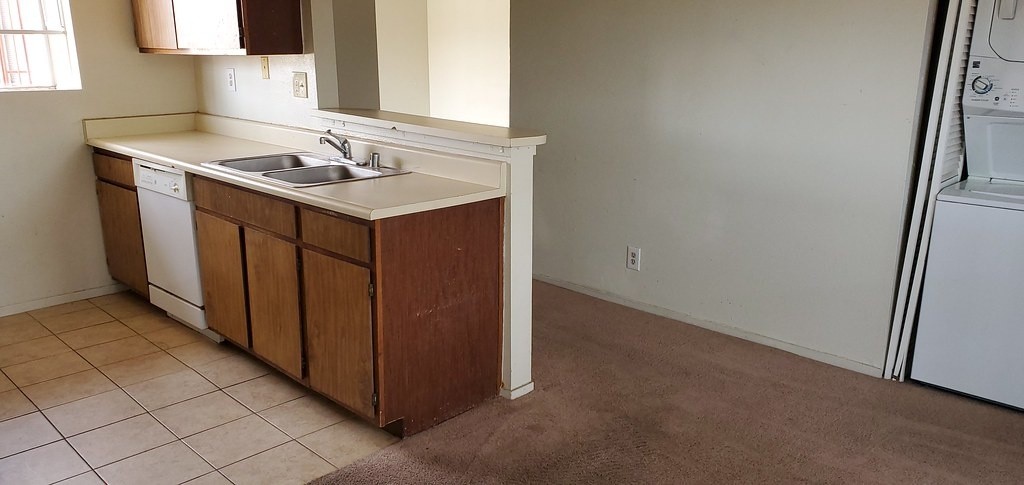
top-left (320, 129), bottom-right (352, 160)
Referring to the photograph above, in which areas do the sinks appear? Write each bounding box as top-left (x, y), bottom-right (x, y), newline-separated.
top-left (200, 151), bottom-right (346, 173)
top-left (260, 164), bottom-right (413, 188)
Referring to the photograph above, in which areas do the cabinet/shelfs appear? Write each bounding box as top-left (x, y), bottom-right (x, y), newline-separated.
top-left (297, 196), bottom-right (506, 438)
top-left (131, 0), bottom-right (302, 56)
top-left (191, 173), bottom-right (310, 390)
top-left (92, 146), bottom-right (149, 301)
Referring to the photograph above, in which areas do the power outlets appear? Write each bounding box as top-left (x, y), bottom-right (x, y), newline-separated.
top-left (291, 72), bottom-right (308, 98)
top-left (261, 57), bottom-right (270, 79)
top-left (626, 245), bottom-right (641, 272)
top-left (226, 68), bottom-right (236, 91)
